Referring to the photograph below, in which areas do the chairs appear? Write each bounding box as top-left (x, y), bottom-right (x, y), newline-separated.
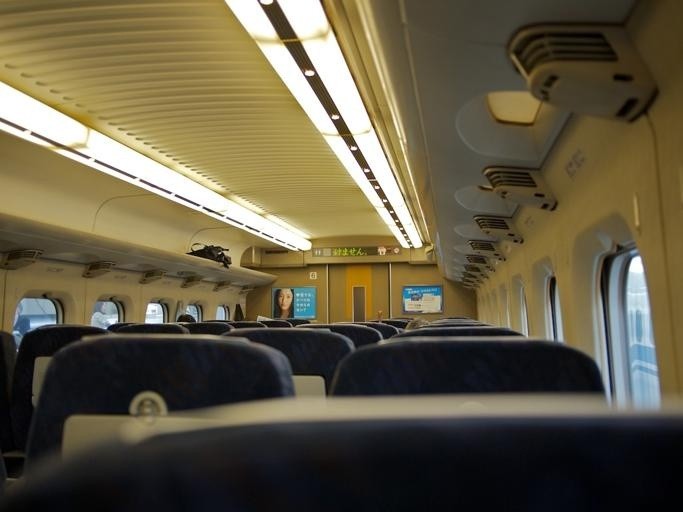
top-left (1, 317), bottom-right (681, 512)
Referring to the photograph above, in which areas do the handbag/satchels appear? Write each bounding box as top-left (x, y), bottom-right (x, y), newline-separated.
top-left (187, 243), bottom-right (232, 269)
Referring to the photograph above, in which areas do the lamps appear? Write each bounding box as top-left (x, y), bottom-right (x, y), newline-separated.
top-left (225, 0), bottom-right (425, 251)
top-left (1, 81), bottom-right (224, 224)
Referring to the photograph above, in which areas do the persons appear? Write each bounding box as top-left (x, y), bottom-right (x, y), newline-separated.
top-left (273, 288), bottom-right (294, 318)
top-left (89, 300), bottom-right (111, 330)
top-left (11, 301), bottom-right (30, 338)
